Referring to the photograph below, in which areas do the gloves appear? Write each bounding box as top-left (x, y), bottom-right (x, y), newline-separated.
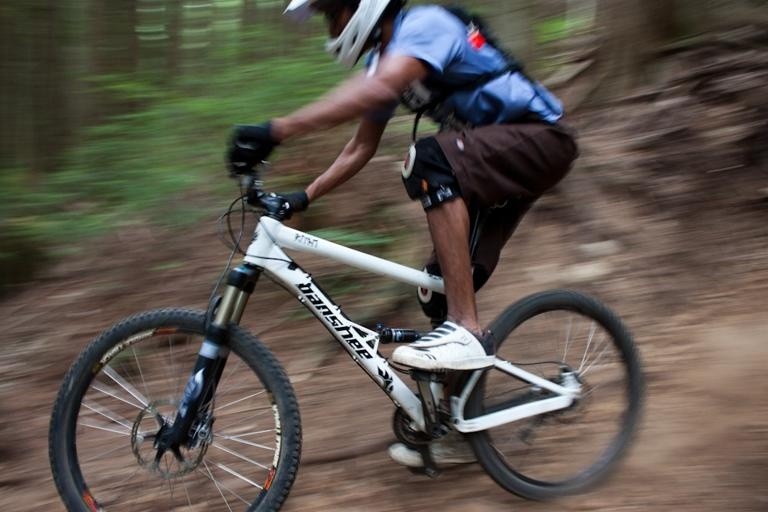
top-left (245, 186), bottom-right (310, 223)
top-left (223, 117), bottom-right (277, 179)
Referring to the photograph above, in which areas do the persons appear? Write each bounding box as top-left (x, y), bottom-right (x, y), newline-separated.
top-left (223, 0), bottom-right (581, 467)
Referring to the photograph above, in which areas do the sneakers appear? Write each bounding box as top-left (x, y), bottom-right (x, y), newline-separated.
top-left (387, 412), bottom-right (483, 467)
top-left (392, 318), bottom-right (497, 372)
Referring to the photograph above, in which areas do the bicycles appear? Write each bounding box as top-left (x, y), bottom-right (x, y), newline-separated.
top-left (46, 125), bottom-right (647, 512)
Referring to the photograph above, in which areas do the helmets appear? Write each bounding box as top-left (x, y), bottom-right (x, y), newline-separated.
top-left (280, 0), bottom-right (391, 72)
